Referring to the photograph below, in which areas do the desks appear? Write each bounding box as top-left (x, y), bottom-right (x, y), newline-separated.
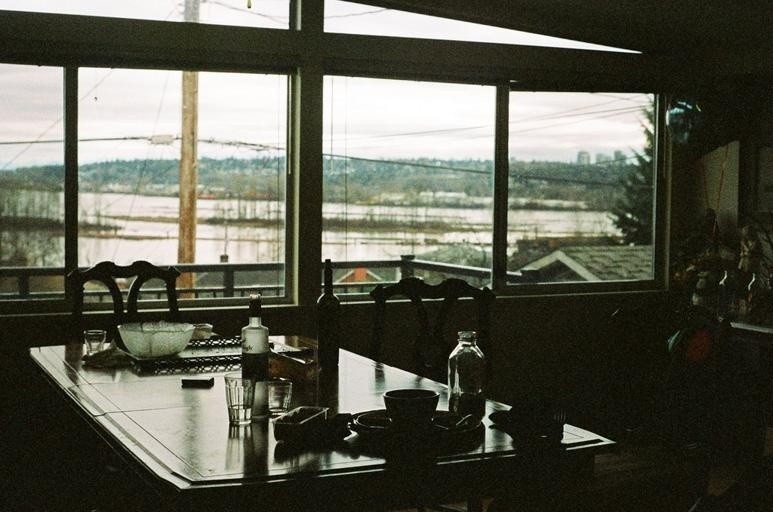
top-left (30, 334), bottom-right (618, 510)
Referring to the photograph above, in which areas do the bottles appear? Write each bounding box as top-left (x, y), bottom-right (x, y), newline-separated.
top-left (317, 367), bottom-right (338, 467)
top-left (238, 292), bottom-right (272, 378)
top-left (312, 258), bottom-right (341, 368)
top-left (446, 329), bottom-right (487, 422)
top-left (243, 416), bottom-right (268, 476)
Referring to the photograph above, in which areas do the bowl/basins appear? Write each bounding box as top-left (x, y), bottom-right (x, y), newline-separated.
top-left (115, 320), bottom-right (196, 359)
top-left (383, 388), bottom-right (441, 422)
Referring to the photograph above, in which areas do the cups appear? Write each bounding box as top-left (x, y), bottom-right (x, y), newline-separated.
top-left (192, 323), bottom-right (212, 339)
top-left (222, 371), bottom-right (255, 424)
top-left (266, 379), bottom-right (294, 416)
top-left (227, 420), bottom-right (255, 471)
top-left (83, 329), bottom-right (105, 356)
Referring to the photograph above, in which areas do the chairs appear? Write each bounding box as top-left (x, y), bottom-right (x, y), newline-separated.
top-left (367, 278), bottom-right (493, 383)
top-left (64, 262), bottom-right (180, 343)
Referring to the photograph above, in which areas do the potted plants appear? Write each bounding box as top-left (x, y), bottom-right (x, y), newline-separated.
top-left (667, 194), bottom-right (771, 363)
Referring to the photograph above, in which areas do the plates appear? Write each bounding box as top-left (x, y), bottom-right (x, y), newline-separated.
top-left (350, 409), bottom-right (481, 439)
